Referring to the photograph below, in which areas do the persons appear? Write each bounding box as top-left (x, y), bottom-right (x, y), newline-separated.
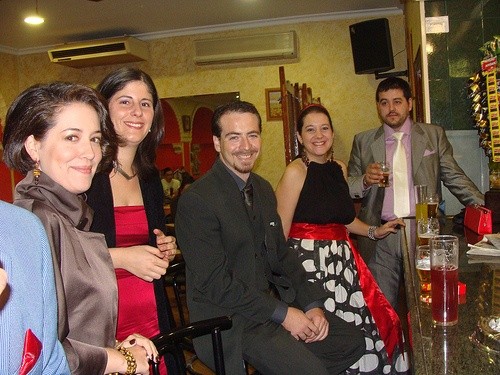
top-left (346, 77), bottom-right (485, 311)
top-left (174, 101), bottom-right (367, 375)
top-left (274, 103), bottom-right (412, 375)
top-left (86, 65), bottom-right (196, 375)
top-left (1, 80), bottom-right (160, 375)
top-left (0, 200), bottom-right (69, 375)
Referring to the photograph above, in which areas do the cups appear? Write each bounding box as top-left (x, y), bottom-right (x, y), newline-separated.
top-left (414, 184), bottom-right (427, 223)
top-left (417, 218), bottom-right (440, 245)
top-left (431, 325), bottom-right (460, 375)
top-left (377, 161), bottom-right (391, 188)
top-left (425, 193), bottom-right (439, 219)
top-left (421, 303), bottom-right (431, 338)
top-left (429, 234), bottom-right (458, 326)
top-left (415, 246), bottom-right (445, 303)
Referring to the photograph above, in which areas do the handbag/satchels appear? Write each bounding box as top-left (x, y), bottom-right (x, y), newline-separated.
top-left (464, 204), bottom-right (492, 235)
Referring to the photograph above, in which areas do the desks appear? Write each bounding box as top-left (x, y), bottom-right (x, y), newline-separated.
top-left (400, 217), bottom-right (500, 375)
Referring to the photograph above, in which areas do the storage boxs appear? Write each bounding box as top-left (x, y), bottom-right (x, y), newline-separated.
top-left (458, 280), bottom-right (467, 295)
top-left (458, 295), bottom-right (467, 304)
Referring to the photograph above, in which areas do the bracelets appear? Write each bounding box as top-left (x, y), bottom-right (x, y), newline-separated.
top-left (363, 179), bottom-right (368, 187)
top-left (108, 346), bottom-right (137, 375)
top-left (368, 225), bottom-right (378, 241)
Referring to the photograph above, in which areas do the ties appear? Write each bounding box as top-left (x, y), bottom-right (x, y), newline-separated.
top-left (241, 186), bottom-right (252, 209)
top-left (391, 132), bottom-right (410, 219)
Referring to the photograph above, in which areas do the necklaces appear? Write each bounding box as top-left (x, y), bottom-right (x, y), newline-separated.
top-left (113, 164), bottom-right (138, 180)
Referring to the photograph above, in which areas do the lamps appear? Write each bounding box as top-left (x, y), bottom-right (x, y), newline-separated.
top-left (24, 0), bottom-right (44, 26)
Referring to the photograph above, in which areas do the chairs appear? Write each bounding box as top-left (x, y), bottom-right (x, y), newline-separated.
top-left (143, 313), bottom-right (233, 375)
top-left (158, 255), bottom-right (260, 375)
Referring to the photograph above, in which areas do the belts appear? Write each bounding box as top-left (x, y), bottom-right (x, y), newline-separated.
top-left (381, 219), bottom-right (401, 230)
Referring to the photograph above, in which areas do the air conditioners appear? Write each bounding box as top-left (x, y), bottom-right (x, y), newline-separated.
top-left (192, 29), bottom-right (298, 66)
top-left (47, 34), bottom-right (149, 69)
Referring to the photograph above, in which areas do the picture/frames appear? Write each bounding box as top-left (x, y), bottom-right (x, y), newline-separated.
top-left (264, 86), bottom-right (312, 121)
top-left (181, 114), bottom-right (191, 133)
